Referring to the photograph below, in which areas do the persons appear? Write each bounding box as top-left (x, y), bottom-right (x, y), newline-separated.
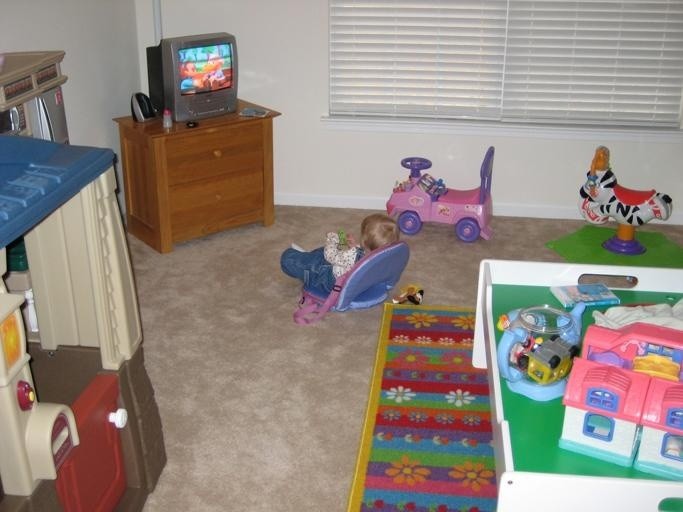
top-left (280, 213), bottom-right (398, 296)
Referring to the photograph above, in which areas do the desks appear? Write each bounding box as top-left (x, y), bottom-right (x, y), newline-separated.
top-left (472, 258), bottom-right (678, 512)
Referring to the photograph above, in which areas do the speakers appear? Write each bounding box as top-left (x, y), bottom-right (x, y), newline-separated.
top-left (131, 92), bottom-right (156, 122)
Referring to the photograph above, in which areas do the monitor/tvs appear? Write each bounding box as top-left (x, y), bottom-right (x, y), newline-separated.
top-left (146, 32), bottom-right (238, 123)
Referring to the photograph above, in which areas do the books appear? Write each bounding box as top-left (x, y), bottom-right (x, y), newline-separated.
top-left (549, 282), bottom-right (620, 309)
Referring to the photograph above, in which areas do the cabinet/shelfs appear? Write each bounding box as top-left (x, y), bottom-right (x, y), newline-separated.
top-left (111, 99), bottom-right (280, 254)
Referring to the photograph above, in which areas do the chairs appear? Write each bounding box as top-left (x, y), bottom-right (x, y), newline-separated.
top-left (304, 241), bottom-right (411, 312)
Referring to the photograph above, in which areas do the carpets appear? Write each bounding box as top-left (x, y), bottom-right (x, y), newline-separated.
top-left (548, 225), bottom-right (681, 269)
top-left (347, 301), bottom-right (501, 511)
